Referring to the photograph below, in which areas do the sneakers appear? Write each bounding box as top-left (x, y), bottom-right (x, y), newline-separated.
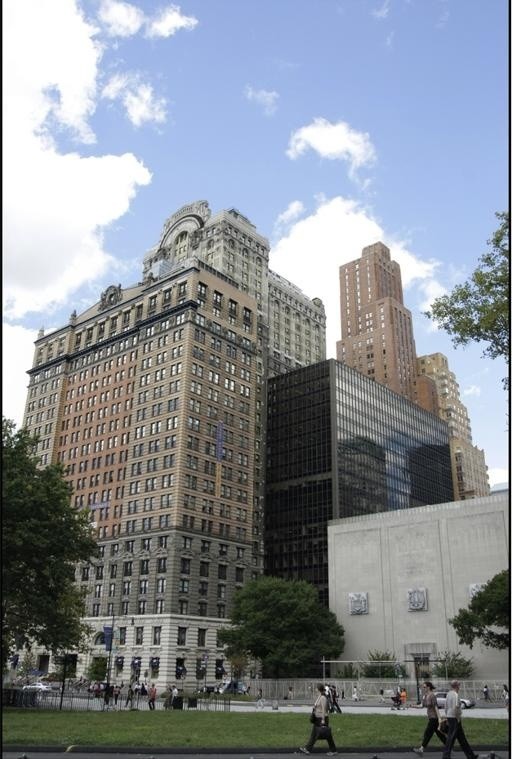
top-left (326, 751), bottom-right (337, 756)
top-left (300, 748), bottom-right (310, 755)
top-left (475, 754), bottom-right (479, 759)
top-left (414, 748), bottom-right (422, 754)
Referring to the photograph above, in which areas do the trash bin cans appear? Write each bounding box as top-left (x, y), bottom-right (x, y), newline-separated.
top-left (173, 696), bottom-right (183, 709)
top-left (272, 700), bottom-right (278, 709)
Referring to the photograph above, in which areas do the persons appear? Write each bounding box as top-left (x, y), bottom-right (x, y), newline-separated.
top-left (413, 681), bottom-right (478, 759)
top-left (94, 683), bottom-right (178, 711)
top-left (483, 684), bottom-right (490, 701)
top-left (380, 688), bottom-right (409, 710)
top-left (300, 683), bottom-right (337, 756)
top-left (256, 689), bottom-right (264, 709)
top-left (501, 684), bottom-right (509, 707)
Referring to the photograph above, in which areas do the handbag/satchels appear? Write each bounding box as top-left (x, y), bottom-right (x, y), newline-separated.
top-left (309, 713), bottom-right (314, 723)
top-left (315, 727), bottom-right (331, 740)
top-left (438, 720), bottom-right (448, 735)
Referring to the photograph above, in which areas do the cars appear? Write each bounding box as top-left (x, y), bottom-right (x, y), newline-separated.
top-left (423, 692), bottom-right (476, 709)
top-left (194, 681), bottom-right (245, 695)
top-left (119, 683), bottom-right (153, 696)
top-left (89, 682), bottom-right (119, 698)
top-left (23, 682), bottom-right (52, 694)
